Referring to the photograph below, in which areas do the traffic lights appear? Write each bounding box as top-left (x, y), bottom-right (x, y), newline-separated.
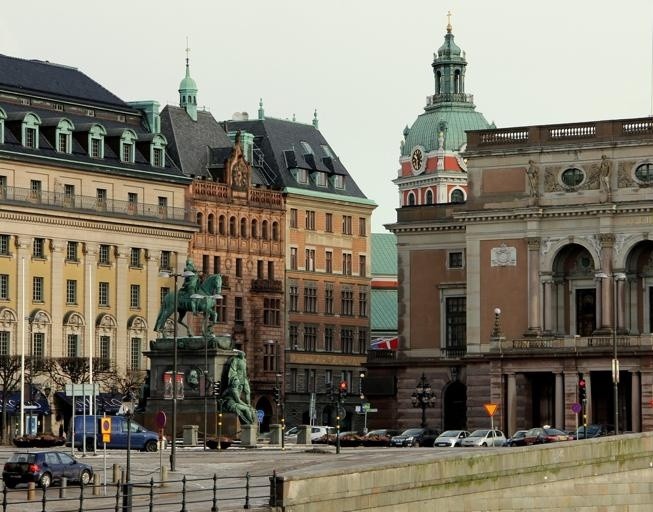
top-left (325, 383), bottom-right (334, 402)
top-left (578, 379), bottom-right (586, 400)
top-left (271, 387), bottom-right (281, 405)
top-left (212, 380), bottom-right (222, 398)
top-left (338, 380), bottom-right (346, 404)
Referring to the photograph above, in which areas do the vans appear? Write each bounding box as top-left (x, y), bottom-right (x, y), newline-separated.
top-left (283, 424), bottom-right (337, 442)
top-left (62, 413), bottom-right (168, 454)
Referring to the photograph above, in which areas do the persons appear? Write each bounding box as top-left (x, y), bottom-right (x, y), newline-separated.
top-left (228, 351), bottom-right (251, 405)
top-left (182, 260), bottom-right (201, 316)
top-left (222, 377), bottom-right (257, 424)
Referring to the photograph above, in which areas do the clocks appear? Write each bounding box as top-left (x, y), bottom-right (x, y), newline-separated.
top-left (410, 145), bottom-right (426, 176)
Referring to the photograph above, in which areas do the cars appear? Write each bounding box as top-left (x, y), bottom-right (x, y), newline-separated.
top-left (504, 429), bottom-right (529, 446)
top-left (362, 429), bottom-right (401, 442)
top-left (332, 429), bottom-right (360, 441)
top-left (508, 424), bottom-right (574, 447)
top-left (2, 450), bottom-right (94, 488)
top-left (570, 423), bottom-right (604, 441)
top-left (432, 430), bottom-right (470, 448)
top-left (459, 429), bottom-right (507, 449)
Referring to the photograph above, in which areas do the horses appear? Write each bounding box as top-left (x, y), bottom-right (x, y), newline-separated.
top-left (153, 272), bottom-right (222, 332)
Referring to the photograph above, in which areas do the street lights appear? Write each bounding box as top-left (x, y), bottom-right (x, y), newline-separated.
top-left (408, 370), bottom-right (438, 427)
top-left (157, 270), bottom-right (198, 475)
top-left (268, 339), bottom-right (280, 424)
top-left (589, 270), bottom-right (626, 436)
top-left (188, 292), bottom-right (223, 452)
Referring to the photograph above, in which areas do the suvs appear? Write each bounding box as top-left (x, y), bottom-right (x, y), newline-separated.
top-left (390, 427), bottom-right (440, 448)
